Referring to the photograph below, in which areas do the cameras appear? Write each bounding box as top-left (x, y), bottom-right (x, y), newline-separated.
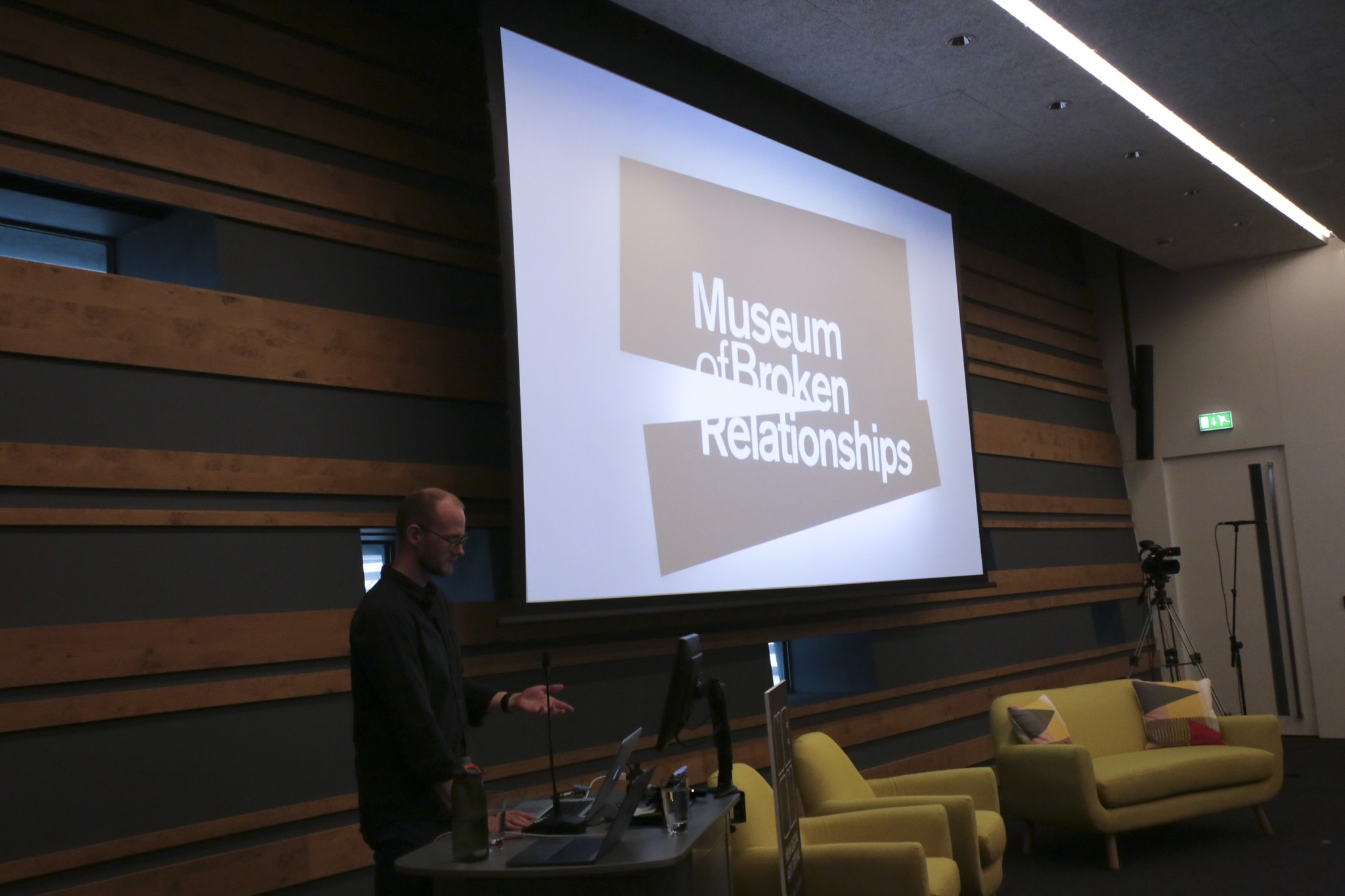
top-left (1138, 540), bottom-right (1181, 575)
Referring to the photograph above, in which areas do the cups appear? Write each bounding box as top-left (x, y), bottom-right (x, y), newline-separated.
top-left (661, 776), bottom-right (690, 832)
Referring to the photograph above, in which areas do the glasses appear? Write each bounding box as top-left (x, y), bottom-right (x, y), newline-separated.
top-left (418, 524), bottom-right (468, 552)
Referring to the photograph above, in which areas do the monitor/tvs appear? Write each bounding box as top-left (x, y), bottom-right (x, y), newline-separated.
top-left (656, 633), bottom-right (738, 799)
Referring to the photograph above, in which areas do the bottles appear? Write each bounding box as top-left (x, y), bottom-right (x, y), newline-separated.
top-left (452, 759), bottom-right (490, 863)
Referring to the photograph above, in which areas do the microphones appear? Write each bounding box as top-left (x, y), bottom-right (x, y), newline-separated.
top-left (1218, 520), bottom-right (1257, 526)
top-left (520, 652), bottom-right (587, 835)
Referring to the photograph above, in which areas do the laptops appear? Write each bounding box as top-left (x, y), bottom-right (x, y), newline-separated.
top-left (534, 727), bottom-right (642, 824)
top-left (505, 765), bottom-right (657, 868)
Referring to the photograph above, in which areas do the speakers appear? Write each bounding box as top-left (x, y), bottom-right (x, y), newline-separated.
top-left (1135, 344), bottom-right (1154, 461)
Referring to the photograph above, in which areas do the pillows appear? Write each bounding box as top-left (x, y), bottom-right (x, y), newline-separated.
top-left (1009, 693), bottom-right (1079, 749)
top-left (1129, 678), bottom-right (1228, 751)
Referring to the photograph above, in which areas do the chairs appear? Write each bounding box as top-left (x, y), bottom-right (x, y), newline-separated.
top-left (790, 729), bottom-right (1007, 896)
top-left (708, 762), bottom-right (962, 896)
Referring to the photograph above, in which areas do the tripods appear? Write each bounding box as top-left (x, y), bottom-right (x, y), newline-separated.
top-left (1125, 575), bottom-right (1227, 716)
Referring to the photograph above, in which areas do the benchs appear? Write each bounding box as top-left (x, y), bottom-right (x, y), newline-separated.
top-left (984, 678), bottom-right (1285, 873)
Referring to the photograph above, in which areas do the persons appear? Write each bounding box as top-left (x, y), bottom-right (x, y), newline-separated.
top-left (350, 486), bottom-right (574, 895)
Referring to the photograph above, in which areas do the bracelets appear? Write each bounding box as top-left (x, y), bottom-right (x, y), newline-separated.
top-left (500, 691), bottom-right (517, 714)
